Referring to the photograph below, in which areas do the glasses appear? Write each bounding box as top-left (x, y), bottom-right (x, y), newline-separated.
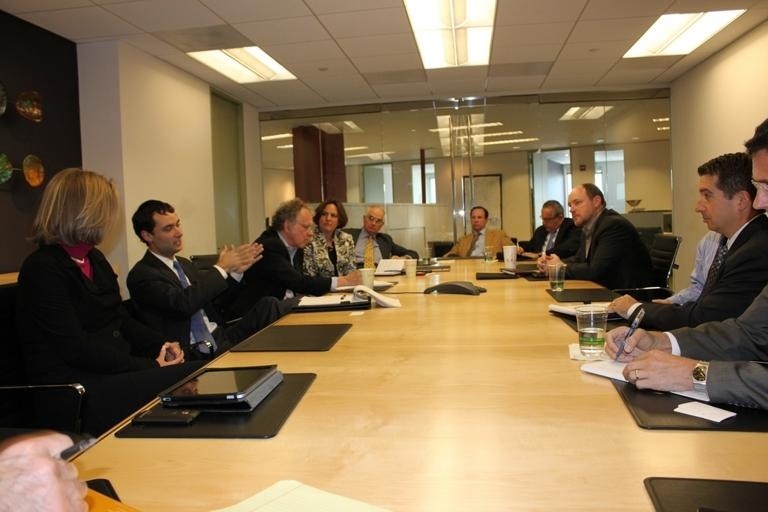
top-left (365, 214), bottom-right (385, 225)
top-left (751, 177), bottom-right (768, 195)
top-left (297, 221), bottom-right (315, 230)
top-left (320, 211), bottom-right (337, 218)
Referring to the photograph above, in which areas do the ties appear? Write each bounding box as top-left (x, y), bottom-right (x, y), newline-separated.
top-left (466, 232), bottom-right (479, 256)
top-left (546, 232), bottom-right (555, 251)
top-left (705, 245), bottom-right (729, 290)
top-left (363, 237), bottom-right (375, 269)
top-left (172, 260), bottom-right (219, 355)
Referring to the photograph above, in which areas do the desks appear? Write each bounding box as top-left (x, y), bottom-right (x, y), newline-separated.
top-left (66, 257), bottom-right (768, 512)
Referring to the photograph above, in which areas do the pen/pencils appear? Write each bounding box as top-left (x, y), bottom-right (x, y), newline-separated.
top-left (516, 240), bottom-right (521, 257)
top-left (61, 437), bottom-right (96, 461)
top-left (614, 308), bottom-right (648, 363)
top-left (341, 294), bottom-right (346, 300)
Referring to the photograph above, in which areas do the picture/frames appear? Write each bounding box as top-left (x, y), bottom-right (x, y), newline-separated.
top-left (462, 174), bottom-right (503, 235)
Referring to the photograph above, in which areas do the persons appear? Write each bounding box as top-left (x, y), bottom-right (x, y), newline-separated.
top-left (516, 200), bottom-right (583, 259)
top-left (651, 230), bottom-right (727, 307)
top-left (1, 430), bottom-right (89, 511)
top-left (126, 199), bottom-right (290, 362)
top-left (608, 153), bottom-right (767, 331)
top-left (302, 198), bottom-right (357, 279)
top-left (341, 202), bottom-right (418, 268)
top-left (604, 119), bottom-right (766, 410)
top-left (442, 205), bottom-right (516, 258)
top-left (239, 197), bottom-right (363, 308)
top-left (538, 183), bottom-right (662, 305)
top-left (10, 166), bottom-right (188, 429)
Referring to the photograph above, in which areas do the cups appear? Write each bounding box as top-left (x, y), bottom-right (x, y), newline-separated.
top-left (571, 304), bottom-right (609, 360)
top-left (420, 247), bottom-right (432, 265)
top-left (403, 259), bottom-right (419, 278)
top-left (358, 268), bottom-right (375, 290)
top-left (502, 244), bottom-right (518, 270)
top-left (484, 246), bottom-right (495, 262)
top-left (546, 262), bottom-right (567, 293)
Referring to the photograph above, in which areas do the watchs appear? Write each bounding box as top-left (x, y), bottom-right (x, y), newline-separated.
top-left (691, 361), bottom-right (709, 392)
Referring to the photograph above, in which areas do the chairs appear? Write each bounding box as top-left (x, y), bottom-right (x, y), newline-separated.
top-left (0, 282), bottom-right (98, 434)
top-left (611, 235), bottom-right (682, 305)
top-left (636, 226), bottom-right (662, 251)
top-left (190, 255), bottom-right (245, 327)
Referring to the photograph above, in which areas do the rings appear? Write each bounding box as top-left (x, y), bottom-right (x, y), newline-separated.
top-left (635, 370), bottom-right (639, 379)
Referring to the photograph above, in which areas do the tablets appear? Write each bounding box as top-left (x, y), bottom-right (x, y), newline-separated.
top-left (157, 365), bottom-right (277, 401)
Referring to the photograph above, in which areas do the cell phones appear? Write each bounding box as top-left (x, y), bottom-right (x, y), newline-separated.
top-left (132, 408), bottom-right (200, 426)
